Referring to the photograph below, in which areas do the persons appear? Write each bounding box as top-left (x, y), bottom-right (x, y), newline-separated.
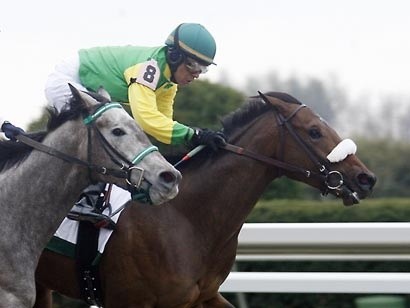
top-left (44, 23), bottom-right (228, 308)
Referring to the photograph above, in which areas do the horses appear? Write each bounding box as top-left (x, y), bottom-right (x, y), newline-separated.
top-left (0, 83), bottom-right (183, 308)
top-left (32, 90), bottom-right (377, 308)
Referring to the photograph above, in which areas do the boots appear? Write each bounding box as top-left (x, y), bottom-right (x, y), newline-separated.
top-left (66, 179), bottom-right (116, 230)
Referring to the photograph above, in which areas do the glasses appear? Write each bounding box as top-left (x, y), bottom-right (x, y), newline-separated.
top-left (184, 55), bottom-right (209, 75)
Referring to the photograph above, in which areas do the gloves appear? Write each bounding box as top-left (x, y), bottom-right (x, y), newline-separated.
top-left (2, 123), bottom-right (27, 142)
top-left (188, 128), bottom-right (229, 155)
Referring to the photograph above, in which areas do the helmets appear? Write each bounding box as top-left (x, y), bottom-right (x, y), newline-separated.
top-left (164, 23), bottom-right (217, 66)
